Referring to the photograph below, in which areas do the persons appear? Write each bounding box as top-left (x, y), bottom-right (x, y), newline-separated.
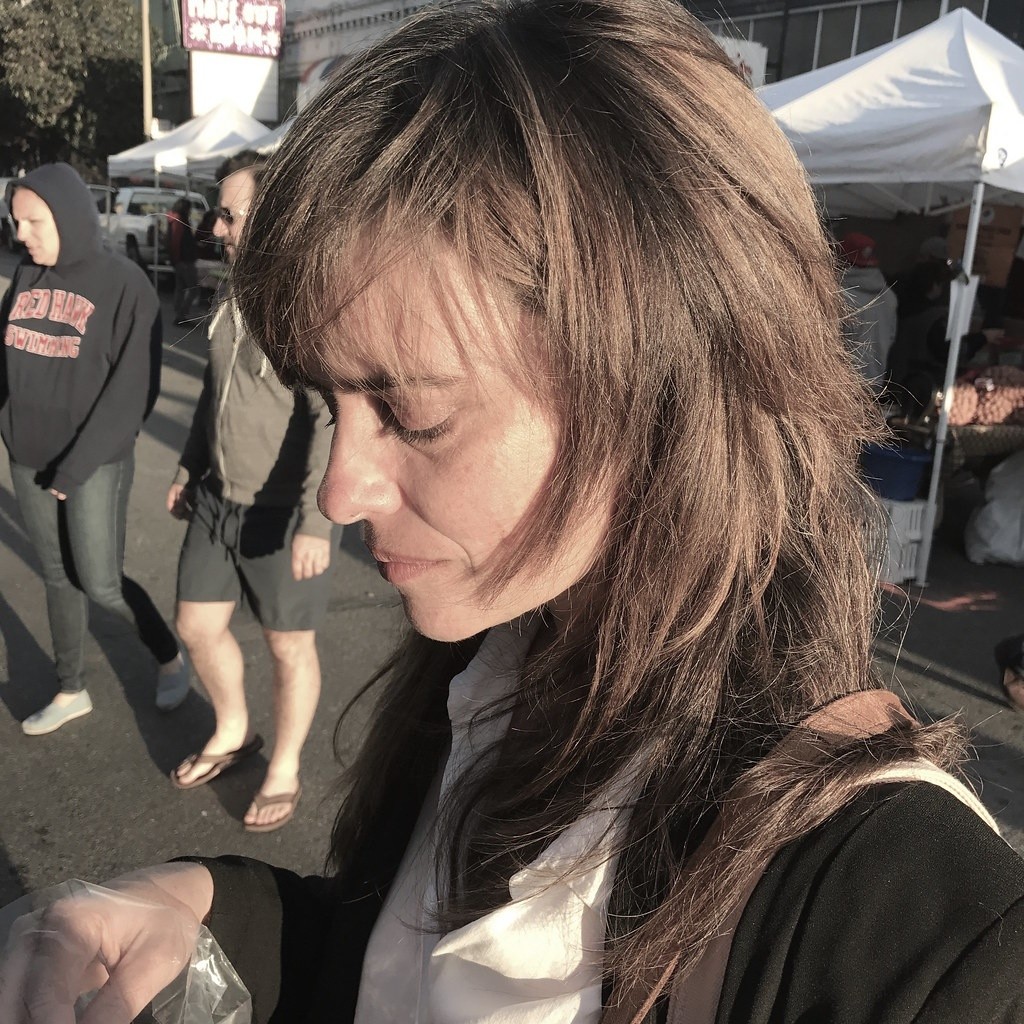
top-left (0, 160), bottom-right (192, 733)
top-left (232, 0), bottom-right (1023, 1024)
top-left (171, 153), bottom-right (336, 837)
top-left (887, 232), bottom-right (999, 419)
top-left (833, 229), bottom-right (898, 398)
top-left (191, 208), bottom-right (226, 308)
top-left (165, 196), bottom-right (201, 327)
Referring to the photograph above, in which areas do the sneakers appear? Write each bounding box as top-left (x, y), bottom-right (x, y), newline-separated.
top-left (155, 654), bottom-right (191, 713)
top-left (22, 689), bottom-right (93, 736)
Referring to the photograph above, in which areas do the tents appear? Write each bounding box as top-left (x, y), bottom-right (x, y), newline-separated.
top-left (746, 7), bottom-right (1023, 584)
top-left (106, 99), bottom-right (313, 298)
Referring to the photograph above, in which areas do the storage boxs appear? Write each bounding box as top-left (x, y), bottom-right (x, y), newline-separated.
top-left (855, 439), bottom-right (930, 585)
top-left (940, 201), bottom-right (1024, 291)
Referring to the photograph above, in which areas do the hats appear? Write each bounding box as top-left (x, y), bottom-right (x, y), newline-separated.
top-left (837, 232), bottom-right (880, 267)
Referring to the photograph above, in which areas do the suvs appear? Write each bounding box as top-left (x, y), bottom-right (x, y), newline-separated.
top-left (97, 186), bottom-right (211, 274)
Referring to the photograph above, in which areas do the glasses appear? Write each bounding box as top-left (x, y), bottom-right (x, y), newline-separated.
top-left (213, 205), bottom-right (250, 225)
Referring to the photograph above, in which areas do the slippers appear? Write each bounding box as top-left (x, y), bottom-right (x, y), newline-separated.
top-left (170, 731), bottom-right (263, 790)
top-left (243, 781), bottom-right (303, 833)
